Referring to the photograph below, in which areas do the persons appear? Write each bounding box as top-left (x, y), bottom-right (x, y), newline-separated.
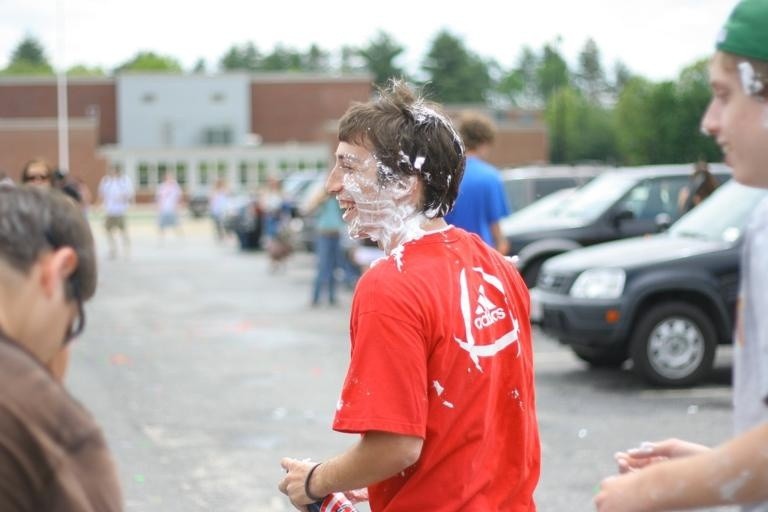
top-left (96, 157), bottom-right (134, 243)
top-left (210, 166), bottom-right (366, 308)
top-left (445, 116), bottom-right (509, 255)
top-left (155, 168), bottom-right (192, 238)
top-left (1, 183), bottom-right (125, 512)
top-left (593, 0), bottom-right (768, 512)
top-left (278, 76), bottom-right (540, 512)
top-left (2, 158), bottom-right (91, 205)
top-left (682, 152), bottom-right (716, 214)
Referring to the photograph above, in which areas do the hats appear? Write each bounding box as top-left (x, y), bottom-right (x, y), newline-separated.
top-left (716, 0), bottom-right (768, 61)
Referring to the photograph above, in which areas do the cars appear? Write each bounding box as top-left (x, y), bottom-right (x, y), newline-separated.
top-left (209, 158), bottom-right (737, 324)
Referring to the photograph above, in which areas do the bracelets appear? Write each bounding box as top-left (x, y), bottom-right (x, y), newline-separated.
top-left (304, 462), bottom-right (331, 501)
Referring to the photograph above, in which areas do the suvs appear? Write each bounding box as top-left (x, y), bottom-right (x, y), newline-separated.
top-left (522, 174), bottom-right (763, 389)
